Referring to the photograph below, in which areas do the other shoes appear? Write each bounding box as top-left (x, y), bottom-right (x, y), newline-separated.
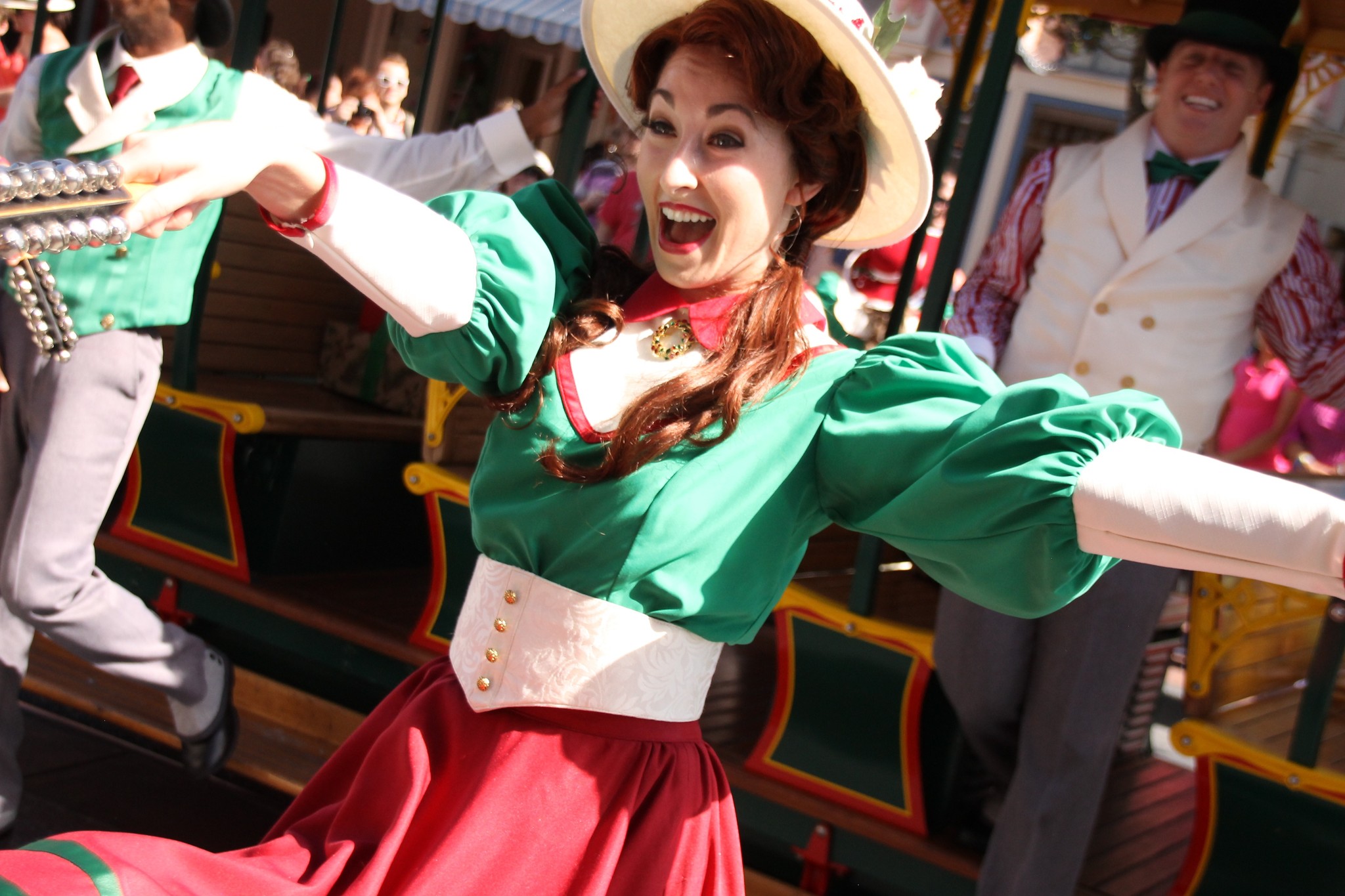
top-left (181, 649), bottom-right (239, 779)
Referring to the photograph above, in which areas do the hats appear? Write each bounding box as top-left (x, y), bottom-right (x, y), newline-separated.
top-left (1144, 0), bottom-right (1304, 91)
top-left (580, 0), bottom-right (933, 250)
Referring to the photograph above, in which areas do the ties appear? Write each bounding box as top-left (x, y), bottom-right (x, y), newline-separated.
top-left (108, 64), bottom-right (138, 108)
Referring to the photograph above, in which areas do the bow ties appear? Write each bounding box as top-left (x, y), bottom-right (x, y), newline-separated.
top-left (1146, 150), bottom-right (1221, 187)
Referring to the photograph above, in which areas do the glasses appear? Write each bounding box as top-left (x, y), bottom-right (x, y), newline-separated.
top-left (377, 77), bottom-right (408, 91)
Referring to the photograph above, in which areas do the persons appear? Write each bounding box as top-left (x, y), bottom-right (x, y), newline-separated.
top-left (929, 4), bottom-right (1343, 896)
top-left (0, 0), bottom-right (1343, 896)
top-left (0, 0), bottom-right (592, 834)
top-left (0, 16), bottom-right (959, 408)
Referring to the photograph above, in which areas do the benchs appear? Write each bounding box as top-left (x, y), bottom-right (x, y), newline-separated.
top-left (1174, 563), bottom-right (1344, 895)
top-left (406, 375), bottom-right (496, 657)
top-left (736, 522), bottom-right (967, 838)
top-left (110, 188), bottom-right (432, 582)
